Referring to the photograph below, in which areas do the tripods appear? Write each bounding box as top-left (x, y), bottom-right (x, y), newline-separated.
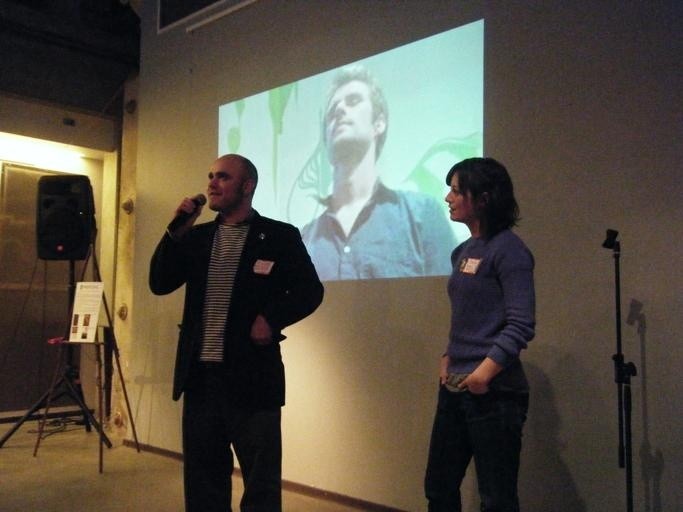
top-left (33, 246), bottom-right (140, 474)
top-left (0, 264), bottom-right (117, 451)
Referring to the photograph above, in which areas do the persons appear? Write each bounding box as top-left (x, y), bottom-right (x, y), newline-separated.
top-left (147, 154), bottom-right (324, 512)
top-left (422, 157), bottom-right (537, 512)
top-left (291, 67), bottom-right (457, 279)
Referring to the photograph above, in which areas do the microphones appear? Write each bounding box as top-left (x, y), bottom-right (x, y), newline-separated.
top-left (165, 194), bottom-right (207, 235)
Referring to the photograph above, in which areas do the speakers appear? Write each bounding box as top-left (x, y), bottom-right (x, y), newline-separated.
top-left (35, 175), bottom-right (94, 262)
